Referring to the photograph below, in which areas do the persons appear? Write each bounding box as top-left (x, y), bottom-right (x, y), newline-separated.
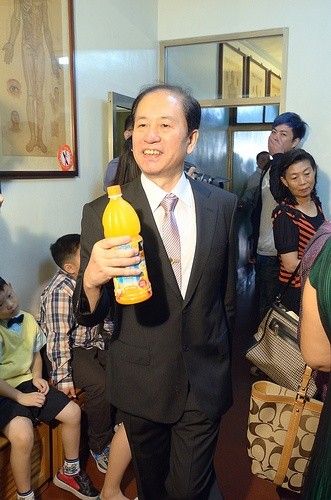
top-left (0, 82), bottom-right (331, 500)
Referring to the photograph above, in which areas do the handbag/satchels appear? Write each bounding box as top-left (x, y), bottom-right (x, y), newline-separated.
top-left (246, 364), bottom-right (324, 493)
top-left (246, 232), bottom-right (331, 398)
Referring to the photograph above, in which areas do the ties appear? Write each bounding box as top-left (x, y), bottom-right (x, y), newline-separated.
top-left (160, 193), bottom-right (181, 294)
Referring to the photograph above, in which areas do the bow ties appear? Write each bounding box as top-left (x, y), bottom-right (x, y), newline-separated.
top-left (8, 314), bottom-right (24, 329)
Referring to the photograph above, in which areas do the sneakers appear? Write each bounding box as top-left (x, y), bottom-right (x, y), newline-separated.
top-left (53, 465), bottom-right (101, 500)
top-left (90, 444), bottom-right (110, 473)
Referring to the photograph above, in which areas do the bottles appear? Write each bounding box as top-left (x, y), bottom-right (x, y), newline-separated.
top-left (101, 185), bottom-right (153, 305)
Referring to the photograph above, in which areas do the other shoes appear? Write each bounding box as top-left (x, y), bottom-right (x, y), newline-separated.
top-left (250, 367), bottom-right (273, 382)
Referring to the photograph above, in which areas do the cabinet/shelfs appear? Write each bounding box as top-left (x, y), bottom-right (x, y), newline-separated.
top-left (0, 374), bottom-right (89, 500)
